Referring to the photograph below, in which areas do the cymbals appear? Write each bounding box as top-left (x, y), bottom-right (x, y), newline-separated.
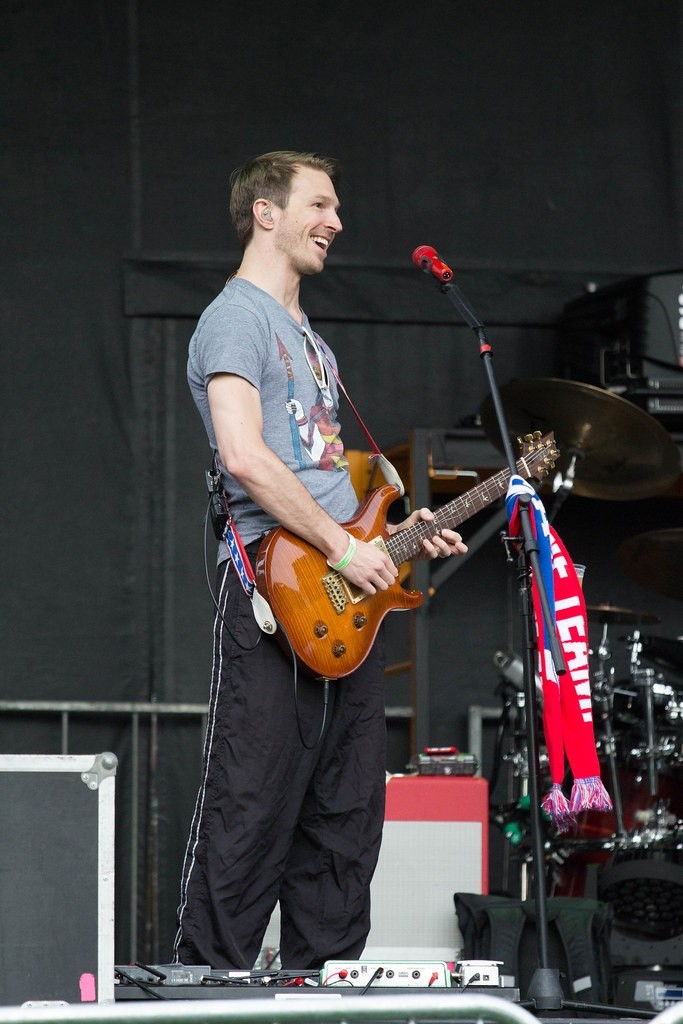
top-left (480, 376), bottom-right (683, 500)
top-left (587, 603), bottom-right (683, 675)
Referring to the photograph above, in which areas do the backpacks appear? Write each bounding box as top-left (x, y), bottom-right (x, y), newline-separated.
top-left (453, 891), bottom-right (614, 1019)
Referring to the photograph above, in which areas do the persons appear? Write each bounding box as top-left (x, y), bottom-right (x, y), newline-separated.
top-left (175, 149), bottom-right (470, 973)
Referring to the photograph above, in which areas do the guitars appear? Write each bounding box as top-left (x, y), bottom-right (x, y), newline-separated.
top-left (255, 429), bottom-right (561, 680)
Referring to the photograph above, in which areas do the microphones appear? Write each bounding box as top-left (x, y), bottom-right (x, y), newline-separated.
top-left (412, 245), bottom-right (453, 283)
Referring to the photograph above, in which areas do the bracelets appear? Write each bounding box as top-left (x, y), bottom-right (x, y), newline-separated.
top-left (327, 532), bottom-right (356, 572)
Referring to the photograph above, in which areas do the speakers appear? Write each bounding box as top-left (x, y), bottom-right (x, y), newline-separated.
top-left (249, 777), bottom-right (490, 971)
top-left (563, 270), bottom-right (683, 435)
top-left (0, 754), bottom-right (117, 1005)
